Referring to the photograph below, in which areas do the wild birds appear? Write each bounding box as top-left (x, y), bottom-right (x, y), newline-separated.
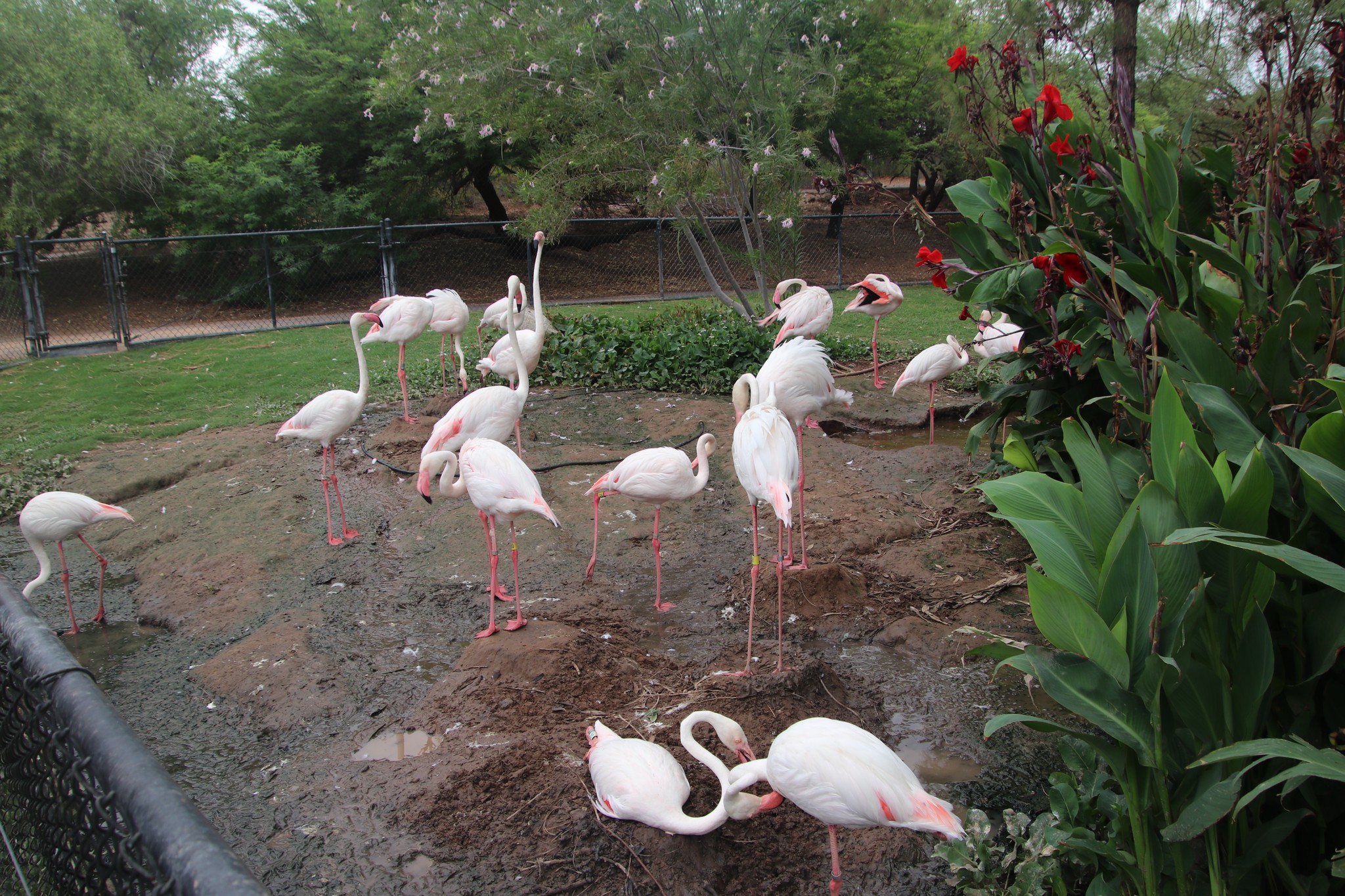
top-left (580, 431), bottom-right (719, 614)
top-left (729, 334), bottom-right (856, 574)
top-left (18, 490), bottom-right (136, 638)
top-left (720, 714), bottom-right (971, 896)
top-left (752, 276), bottom-right (835, 430)
top-left (414, 434), bottom-right (563, 641)
top-left (419, 273), bottom-right (532, 603)
top-left (273, 311), bottom-right (385, 546)
top-left (891, 333), bottom-right (970, 446)
top-left (423, 285), bottom-right (469, 398)
top-left (838, 272), bottom-right (905, 389)
top-left (474, 230), bottom-right (547, 475)
top-left (581, 708), bottom-right (758, 837)
top-left (722, 402), bottom-right (801, 677)
top-left (475, 281), bottom-right (528, 390)
top-left (972, 307), bottom-right (1026, 446)
top-left (358, 294), bottom-right (435, 425)
